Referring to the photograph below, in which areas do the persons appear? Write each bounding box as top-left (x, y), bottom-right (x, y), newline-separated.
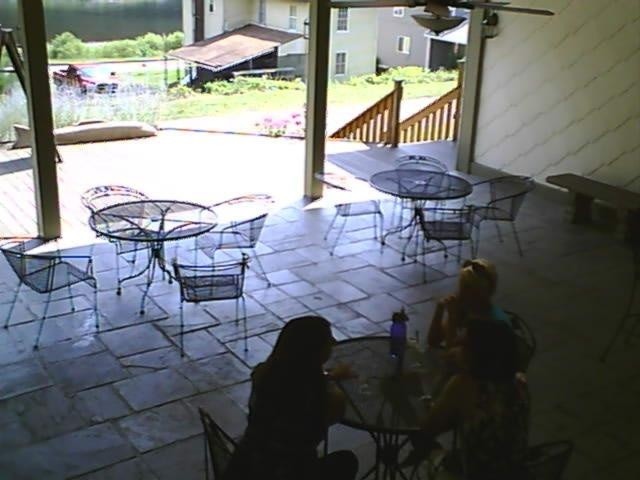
top-left (222, 315), bottom-right (360, 480)
top-left (420, 313), bottom-right (531, 479)
top-left (388, 258), bottom-right (499, 469)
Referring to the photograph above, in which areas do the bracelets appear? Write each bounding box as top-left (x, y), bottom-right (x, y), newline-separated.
top-left (419, 395), bottom-right (433, 402)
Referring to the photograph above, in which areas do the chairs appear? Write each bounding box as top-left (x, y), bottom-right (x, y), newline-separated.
top-left (599, 211), bottom-right (640, 362)
top-left (503, 311), bottom-right (535, 373)
top-left (524, 440), bottom-right (573, 480)
top-left (313, 155), bottom-right (536, 285)
top-left (198, 406), bottom-right (240, 480)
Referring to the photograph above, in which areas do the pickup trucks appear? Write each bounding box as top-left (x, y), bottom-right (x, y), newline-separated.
top-left (53, 64), bottom-right (120, 97)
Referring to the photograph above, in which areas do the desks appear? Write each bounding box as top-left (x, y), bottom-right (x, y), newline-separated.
top-left (322, 335), bottom-right (458, 480)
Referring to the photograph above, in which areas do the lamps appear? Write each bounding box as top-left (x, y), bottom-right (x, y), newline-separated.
top-left (411, 6), bottom-right (466, 34)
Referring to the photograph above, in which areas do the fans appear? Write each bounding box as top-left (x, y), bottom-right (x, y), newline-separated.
top-left (329, 0), bottom-right (555, 17)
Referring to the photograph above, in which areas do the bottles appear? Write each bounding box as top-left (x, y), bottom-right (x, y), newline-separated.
top-left (391, 307), bottom-right (409, 361)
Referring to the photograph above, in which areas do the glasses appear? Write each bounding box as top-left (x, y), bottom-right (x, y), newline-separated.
top-left (463, 261), bottom-right (492, 276)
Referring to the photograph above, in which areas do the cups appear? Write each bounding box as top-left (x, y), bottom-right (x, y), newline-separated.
top-left (416, 331), bottom-right (429, 353)
